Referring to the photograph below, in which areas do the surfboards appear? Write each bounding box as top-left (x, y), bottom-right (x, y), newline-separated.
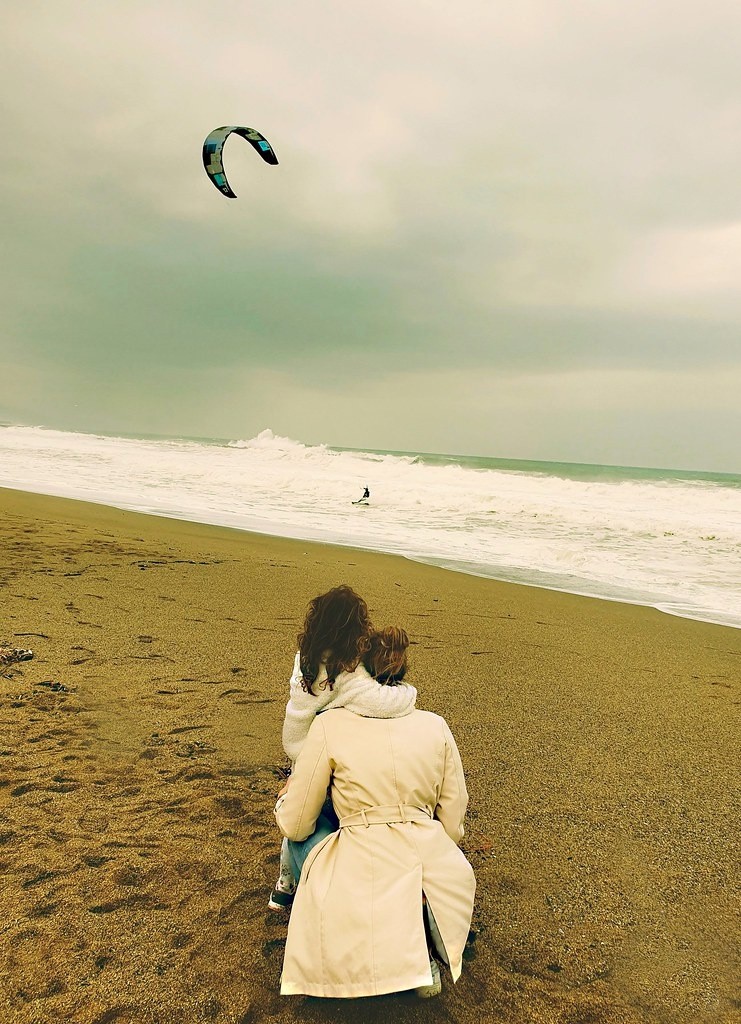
top-left (351, 501), bottom-right (371, 506)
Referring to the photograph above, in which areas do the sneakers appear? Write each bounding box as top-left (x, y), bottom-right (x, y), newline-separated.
top-left (267, 888), bottom-right (297, 912)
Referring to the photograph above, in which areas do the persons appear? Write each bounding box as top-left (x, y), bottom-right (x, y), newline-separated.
top-left (351, 487), bottom-right (370, 506)
top-left (266, 584), bottom-right (478, 997)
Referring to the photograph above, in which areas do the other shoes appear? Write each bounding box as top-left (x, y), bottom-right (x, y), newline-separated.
top-left (414, 954), bottom-right (442, 999)
